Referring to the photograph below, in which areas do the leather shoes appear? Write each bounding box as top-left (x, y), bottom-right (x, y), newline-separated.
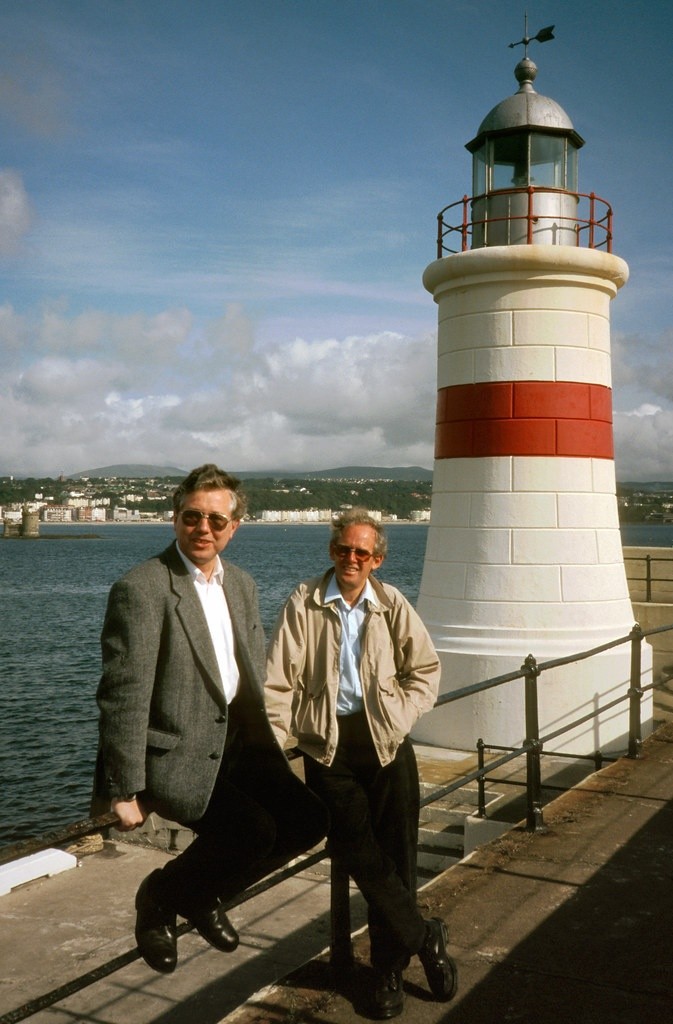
top-left (135, 870), bottom-right (177, 973)
top-left (420, 918), bottom-right (458, 1002)
top-left (361, 965), bottom-right (404, 1019)
top-left (180, 890), bottom-right (239, 952)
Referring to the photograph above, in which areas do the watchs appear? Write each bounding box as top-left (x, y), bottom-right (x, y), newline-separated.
top-left (118, 793), bottom-right (136, 801)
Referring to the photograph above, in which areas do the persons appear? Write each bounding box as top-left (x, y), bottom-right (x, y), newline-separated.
top-left (264, 508), bottom-right (457, 1019)
top-left (90, 465), bottom-right (327, 973)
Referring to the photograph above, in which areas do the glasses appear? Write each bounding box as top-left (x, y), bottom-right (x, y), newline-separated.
top-left (331, 540), bottom-right (374, 561)
top-left (176, 504), bottom-right (238, 531)
